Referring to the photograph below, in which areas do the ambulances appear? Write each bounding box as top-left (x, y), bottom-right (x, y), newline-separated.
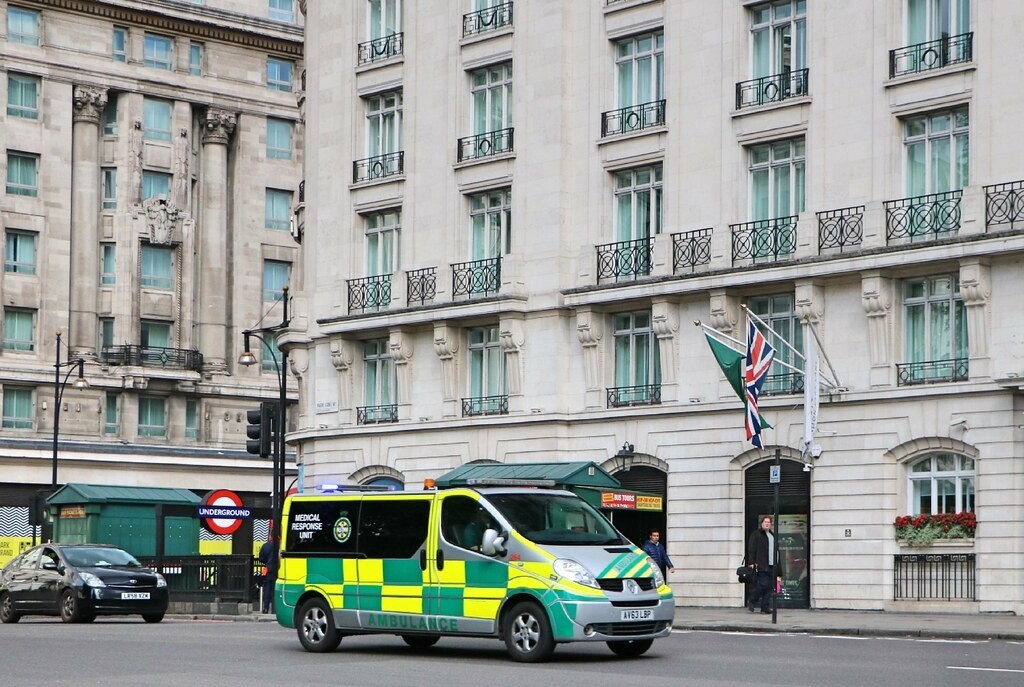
top-left (274, 488), bottom-right (674, 662)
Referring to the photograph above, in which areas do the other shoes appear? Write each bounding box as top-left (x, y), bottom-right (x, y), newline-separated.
top-left (761, 607), bottom-right (773, 614)
top-left (262, 609), bottom-right (268, 613)
top-left (747, 600), bottom-right (754, 612)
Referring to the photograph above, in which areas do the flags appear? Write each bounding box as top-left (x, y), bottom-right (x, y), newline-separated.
top-left (701, 308), bottom-right (779, 451)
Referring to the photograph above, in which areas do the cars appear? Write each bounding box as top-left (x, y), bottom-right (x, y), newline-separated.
top-left (1, 541), bottom-right (168, 623)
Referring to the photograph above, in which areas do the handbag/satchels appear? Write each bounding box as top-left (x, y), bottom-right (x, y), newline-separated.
top-left (737, 564), bottom-right (758, 583)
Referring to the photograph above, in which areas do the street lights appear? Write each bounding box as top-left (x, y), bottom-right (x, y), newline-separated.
top-left (52, 330), bottom-right (90, 493)
top-left (237, 286), bottom-right (291, 504)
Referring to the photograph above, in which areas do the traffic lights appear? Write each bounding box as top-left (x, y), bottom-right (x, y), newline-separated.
top-left (247, 402), bottom-right (271, 458)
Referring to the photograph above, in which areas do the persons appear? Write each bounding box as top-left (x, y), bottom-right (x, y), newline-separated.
top-left (259, 531), bottom-right (274, 614)
top-left (463, 501), bottom-right (500, 549)
top-left (642, 530), bottom-right (675, 573)
top-left (745, 515), bottom-right (783, 614)
